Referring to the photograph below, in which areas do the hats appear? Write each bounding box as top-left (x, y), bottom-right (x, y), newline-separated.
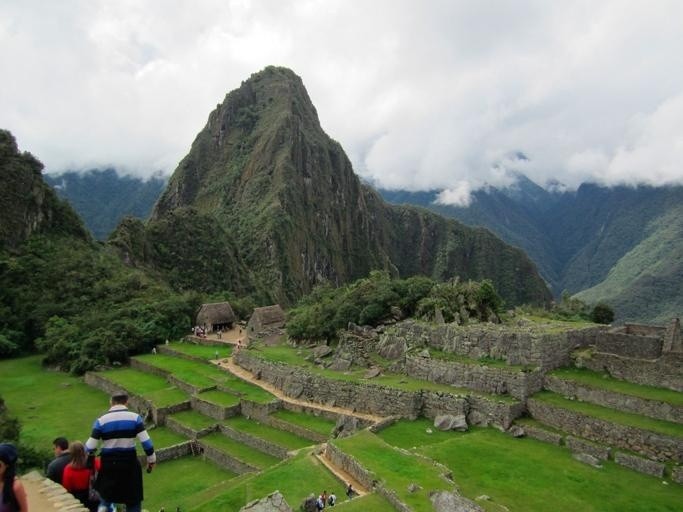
top-left (0, 444), bottom-right (19, 465)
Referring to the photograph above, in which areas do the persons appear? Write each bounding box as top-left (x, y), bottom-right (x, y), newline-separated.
top-left (174, 506), bottom-right (182, 512)
top-left (0, 444), bottom-right (28, 511)
top-left (320, 489), bottom-right (327, 509)
top-left (345, 483), bottom-right (355, 501)
top-left (189, 325), bottom-right (222, 340)
top-left (158, 506), bottom-right (164, 512)
top-left (315, 494), bottom-right (324, 512)
top-left (327, 491), bottom-right (336, 506)
top-left (83, 390), bottom-right (156, 512)
top-left (215, 349), bottom-right (218, 360)
top-left (43, 436), bottom-right (73, 486)
top-left (63, 438), bottom-right (99, 500)
